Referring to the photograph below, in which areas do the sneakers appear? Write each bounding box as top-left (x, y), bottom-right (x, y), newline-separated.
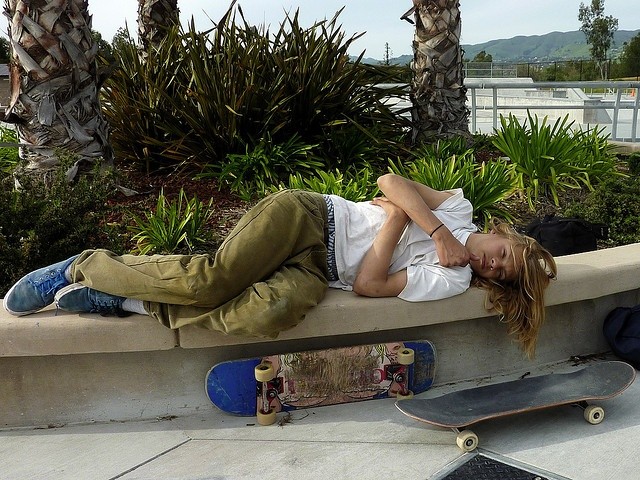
top-left (54, 282), bottom-right (132, 318)
top-left (3, 253), bottom-right (78, 317)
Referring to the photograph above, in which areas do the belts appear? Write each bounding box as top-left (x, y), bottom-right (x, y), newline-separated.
top-left (324, 193), bottom-right (338, 282)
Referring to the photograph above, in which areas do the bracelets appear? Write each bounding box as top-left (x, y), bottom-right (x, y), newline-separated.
top-left (429, 222), bottom-right (446, 240)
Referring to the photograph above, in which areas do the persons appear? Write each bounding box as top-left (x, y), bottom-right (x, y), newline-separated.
top-left (4, 173), bottom-right (558, 363)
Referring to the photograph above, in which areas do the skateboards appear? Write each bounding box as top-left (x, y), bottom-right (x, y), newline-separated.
top-left (207, 340), bottom-right (438, 425)
top-left (395, 361), bottom-right (636, 451)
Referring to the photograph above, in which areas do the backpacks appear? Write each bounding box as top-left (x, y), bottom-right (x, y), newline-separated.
top-left (525, 212), bottom-right (608, 258)
